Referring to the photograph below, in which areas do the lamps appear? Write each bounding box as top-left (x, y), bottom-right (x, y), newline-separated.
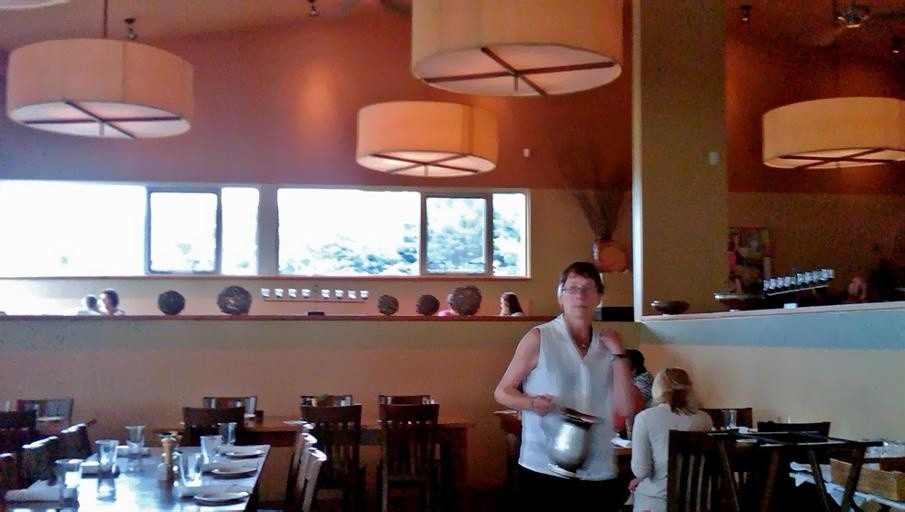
top-left (6, 2), bottom-right (196, 139)
top-left (761, 2), bottom-right (905, 171)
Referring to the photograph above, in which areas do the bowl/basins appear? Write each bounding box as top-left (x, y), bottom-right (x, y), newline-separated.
top-left (650, 300), bottom-right (690, 315)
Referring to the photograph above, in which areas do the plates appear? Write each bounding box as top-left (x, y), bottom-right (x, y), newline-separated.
top-left (192, 445), bottom-right (265, 505)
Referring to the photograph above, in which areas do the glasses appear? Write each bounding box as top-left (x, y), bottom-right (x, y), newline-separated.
top-left (562, 283), bottom-right (598, 295)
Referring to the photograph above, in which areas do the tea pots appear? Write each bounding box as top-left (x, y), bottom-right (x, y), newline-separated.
top-left (538, 403), bottom-right (606, 479)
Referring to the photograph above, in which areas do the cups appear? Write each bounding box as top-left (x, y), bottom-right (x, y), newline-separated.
top-left (53, 422), bottom-right (237, 508)
top-left (722, 408), bottom-right (738, 430)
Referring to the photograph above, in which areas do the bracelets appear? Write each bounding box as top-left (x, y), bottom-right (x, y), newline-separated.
top-left (531, 399), bottom-right (535, 409)
top-left (609, 353), bottom-right (626, 361)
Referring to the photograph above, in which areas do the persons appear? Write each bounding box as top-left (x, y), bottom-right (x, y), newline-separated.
top-left (98, 288), bottom-right (125, 316)
top-left (494, 263), bottom-right (634, 512)
top-left (499, 292), bottom-right (526, 317)
top-left (76, 295), bottom-right (103, 316)
top-left (438, 294), bottom-right (459, 317)
top-left (613, 349), bottom-right (654, 439)
top-left (627, 367), bottom-right (713, 512)
